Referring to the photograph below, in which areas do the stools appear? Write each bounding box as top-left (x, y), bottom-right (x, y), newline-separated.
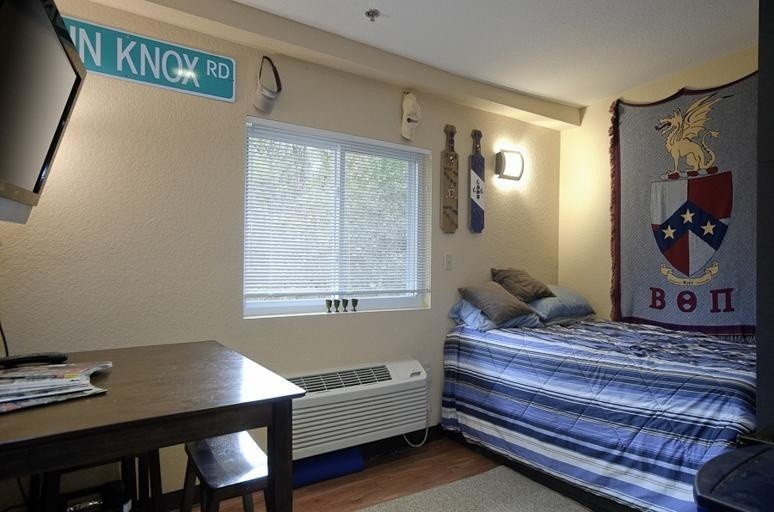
top-left (179, 430), bottom-right (268, 511)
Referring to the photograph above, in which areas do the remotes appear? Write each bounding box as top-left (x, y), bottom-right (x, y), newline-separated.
top-left (0, 350), bottom-right (70, 368)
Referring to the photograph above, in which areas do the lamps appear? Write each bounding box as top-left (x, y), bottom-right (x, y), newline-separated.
top-left (495, 150), bottom-right (524, 181)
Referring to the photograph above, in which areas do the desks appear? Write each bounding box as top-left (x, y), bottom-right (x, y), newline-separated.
top-left (0, 340), bottom-right (306, 511)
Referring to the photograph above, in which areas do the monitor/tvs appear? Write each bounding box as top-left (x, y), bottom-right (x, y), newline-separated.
top-left (0, 0), bottom-right (88, 208)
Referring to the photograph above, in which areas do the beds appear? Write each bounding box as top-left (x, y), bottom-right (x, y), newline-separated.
top-left (439, 318), bottom-right (759, 511)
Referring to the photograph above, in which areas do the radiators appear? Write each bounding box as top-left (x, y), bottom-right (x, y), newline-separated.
top-left (284, 357), bottom-right (427, 462)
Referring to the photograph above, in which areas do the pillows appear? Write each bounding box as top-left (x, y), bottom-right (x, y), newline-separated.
top-left (457, 281), bottom-right (534, 325)
top-left (491, 268), bottom-right (556, 303)
top-left (527, 284), bottom-right (594, 321)
top-left (447, 297), bottom-right (546, 332)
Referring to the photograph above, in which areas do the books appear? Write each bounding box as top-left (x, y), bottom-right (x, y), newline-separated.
top-left (0, 361), bottom-right (114, 415)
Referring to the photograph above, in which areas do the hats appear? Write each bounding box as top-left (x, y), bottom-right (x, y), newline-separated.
top-left (402, 91), bottom-right (422, 139)
top-left (253, 55), bottom-right (281, 112)
